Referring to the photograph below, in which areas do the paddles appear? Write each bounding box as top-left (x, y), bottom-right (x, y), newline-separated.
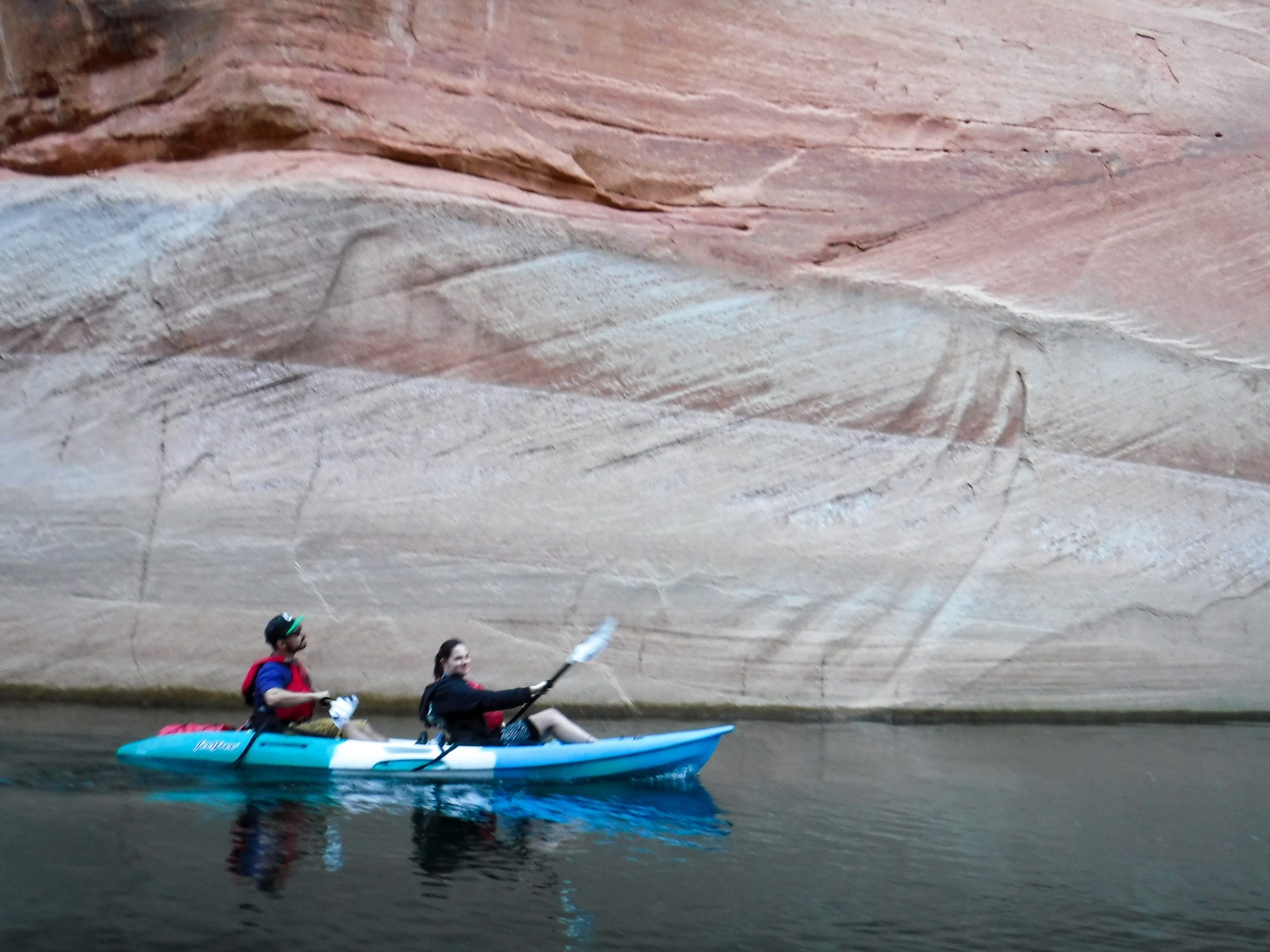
top-left (505, 617), bottom-right (619, 732)
top-left (321, 693), bottom-right (360, 734)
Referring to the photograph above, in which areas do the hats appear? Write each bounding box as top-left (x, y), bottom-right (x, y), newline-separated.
top-left (264, 612), bottom-right (304, 642)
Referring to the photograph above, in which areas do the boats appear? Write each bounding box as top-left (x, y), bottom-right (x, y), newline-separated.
top-left (117, 724), bottom-right (735, 781)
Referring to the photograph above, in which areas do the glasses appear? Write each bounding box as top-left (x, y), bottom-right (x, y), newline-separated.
top-left (287, 626), bottom-right (303, 637)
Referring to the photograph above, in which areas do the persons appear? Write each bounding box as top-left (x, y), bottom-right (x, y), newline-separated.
top-left (241, 611), bottom-right (385, 742)
top-left (419, 638), bottom-right (598, 746)
